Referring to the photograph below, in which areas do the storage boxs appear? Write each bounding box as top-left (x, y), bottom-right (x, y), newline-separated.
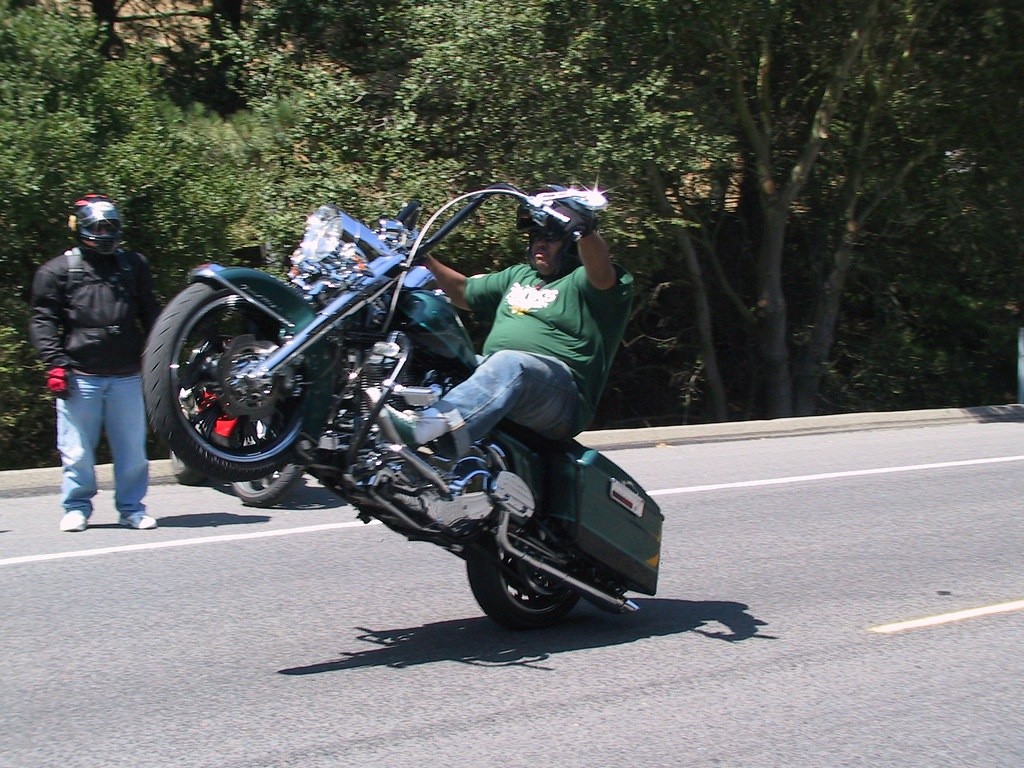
top-left (549, 441), bottom-right (667, 597)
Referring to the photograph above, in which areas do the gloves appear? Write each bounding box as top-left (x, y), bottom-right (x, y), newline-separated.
top-left (46, 368), bottom-right (74, 401)
top-left (545, 206), bottom-right (593, 240)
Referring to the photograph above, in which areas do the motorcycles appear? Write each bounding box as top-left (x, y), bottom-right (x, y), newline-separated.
top-left (141, 177), bottom-right (664, 631)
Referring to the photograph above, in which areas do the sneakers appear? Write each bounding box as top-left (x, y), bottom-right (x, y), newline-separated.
top-left (364, 387), bottom-right (450, 453)
top-left (118, 510), bottom-right (157, 529)
top-left (59, 510), bottom-right (88, 531)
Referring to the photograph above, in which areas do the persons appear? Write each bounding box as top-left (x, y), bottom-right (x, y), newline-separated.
top-left (28, 194), bottom-right (163, 532)
top-left (366, 191), bottom-right (634, 453)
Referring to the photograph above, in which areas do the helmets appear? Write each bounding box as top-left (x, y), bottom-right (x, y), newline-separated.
top-left (515, 184), bottom-right (598, 290)
top-left (66, 194), bottom-right (122, 256)
